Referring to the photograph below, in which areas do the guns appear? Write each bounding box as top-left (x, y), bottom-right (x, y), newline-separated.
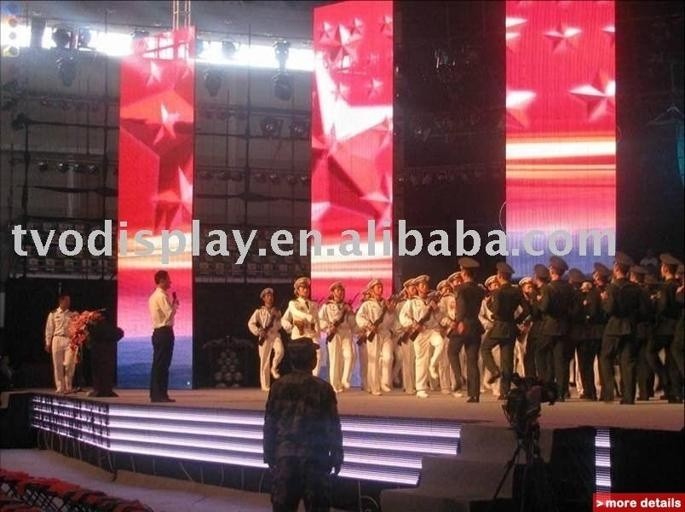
top-left (357, 284), bottom-right (447, 345)
top-left (325, 291), bottom-right (360, 342)
top-left (256, 292), bottom-right (289, 346)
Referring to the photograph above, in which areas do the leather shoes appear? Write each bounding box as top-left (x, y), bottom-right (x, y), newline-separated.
top-left (322, 369), bottom-right (684, 406)
top-left (270, 366), bottom-right (284, 381)
top-left (150, 398), bottom-right (177, 403)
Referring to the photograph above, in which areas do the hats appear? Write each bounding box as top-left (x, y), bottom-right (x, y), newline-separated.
top-left (457, 255), bottom-right (481, 272)
top-left (594, 261), bottom-right (611, 278)
top-left (328, 281), bottom-right (342, 292)
top-left (293, 276), bottom-right (312, 290)
top-left (414, 273), bottom-right (432, 284)
top-left (495, 261), bottom-right (515, 277)
top-left (448, 271), bottom-right (462, 282)
top-left (569, 267), bottom-right (586, 285)
top-left (517, 276), bottom-right (532, 286)
top-left (630, 262), bottom-right (650, 277)
top-left (484, 274), bottom-right (498, 286)
top-left (549, 254), bottom-right (570, 272)
top-left (534, 264), bottom-right (551, 283)
top-left (614, 251), bottom-right (636, 271)
top-left (436, 279), bottom-right (449, 290)
top-left (258, 287), bottom-right (274, 299)
top-left (365, 278), bottom-right (385, 289)
top-left (286, 337), bottom-right (320, 360)
top-left (659, 250), bottom-right (682, 268)
top-left (403, 277), bottom-right (415, 287)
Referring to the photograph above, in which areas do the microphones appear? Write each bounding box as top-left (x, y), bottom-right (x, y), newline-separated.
top-left (173, 291), bottom-right (179, 306)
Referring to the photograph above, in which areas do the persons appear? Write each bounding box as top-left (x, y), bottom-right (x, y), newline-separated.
top-left (44, 291), bottom-right (80, 392)
top-left (148, 270), bottom-right (180, 402)
top-left (0, 350), bottom-right (16, 389)
top-left (264, 338), bottom-right (344, 512)
top-left (247, 287), bottom-right (284, 393)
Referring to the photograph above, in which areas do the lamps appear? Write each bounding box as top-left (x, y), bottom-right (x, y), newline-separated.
top-left (0, 21), bottom-right (506, 188)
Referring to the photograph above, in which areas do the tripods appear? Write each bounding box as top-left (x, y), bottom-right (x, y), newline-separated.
top-left (491, 434), bottom-right (560, 512)
